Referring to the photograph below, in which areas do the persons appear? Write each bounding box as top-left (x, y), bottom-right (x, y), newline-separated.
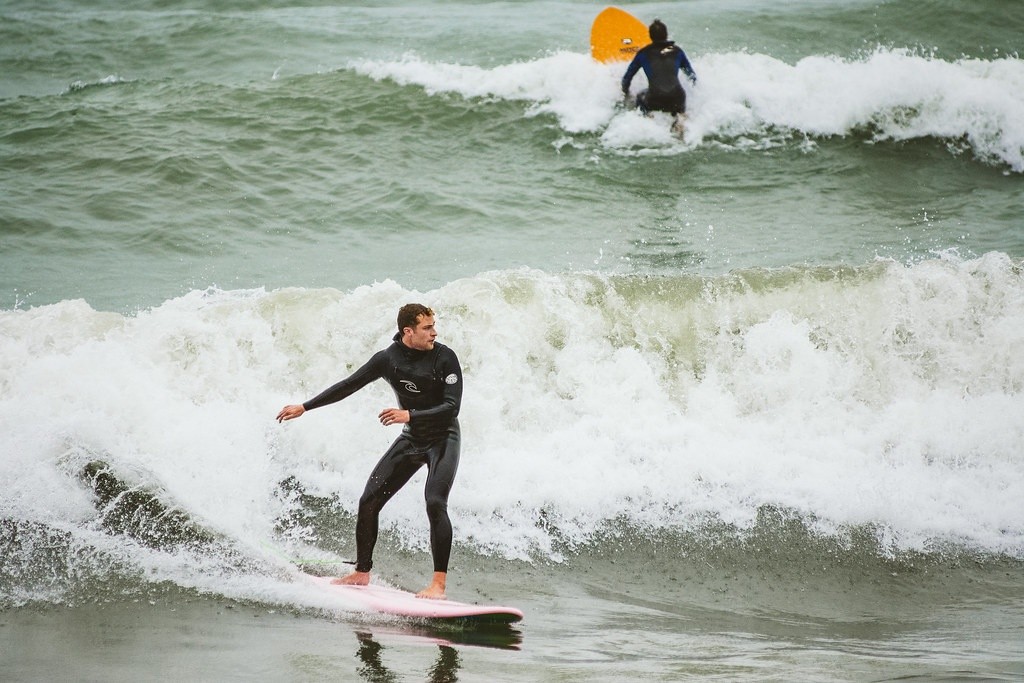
top-left (622, 18), bottom-right (697, 135)
top-left (276, 304), bottom-right (463, 600)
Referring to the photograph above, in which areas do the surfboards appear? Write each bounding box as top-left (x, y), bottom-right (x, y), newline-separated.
top-left (324, 576), bottom-right (523, 626)
top-left (590, 6), bottom-right (654, 63)
